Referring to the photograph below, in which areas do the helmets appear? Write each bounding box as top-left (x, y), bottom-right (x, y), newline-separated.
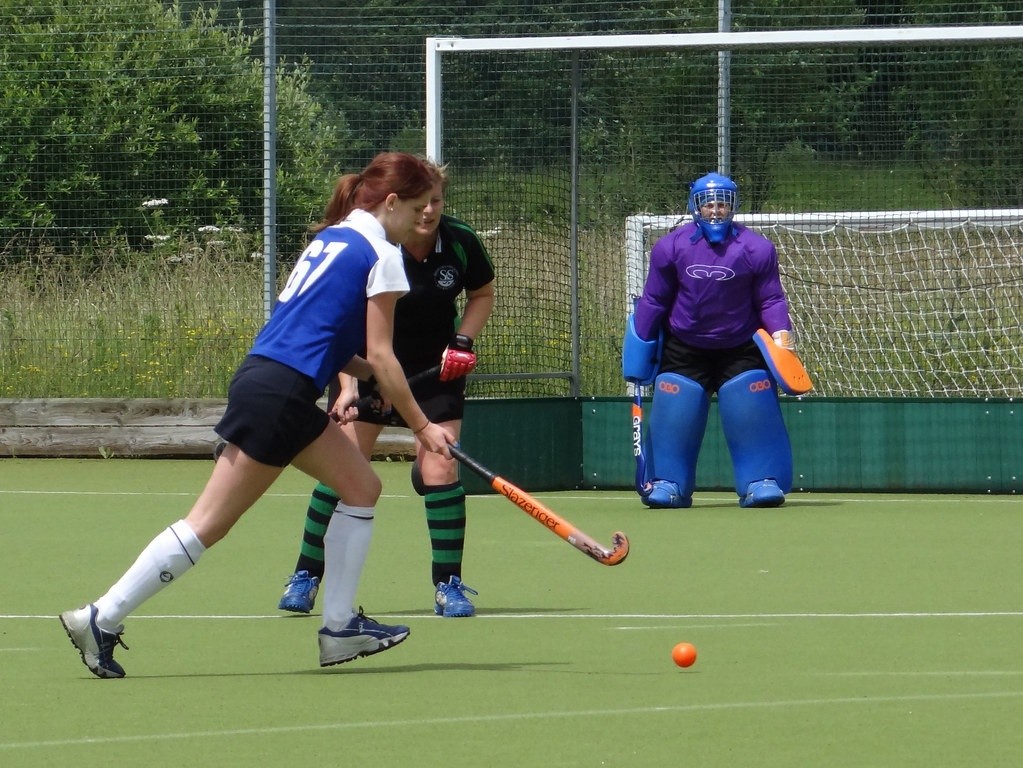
top-left (689, 173), bottom-right (741, 245)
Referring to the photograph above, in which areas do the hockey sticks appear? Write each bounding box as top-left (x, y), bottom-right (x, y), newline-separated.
top-left (214, 364), bottom-right (440, 466)
top-left (445, 440), bottom-right (629, 566)
top-left (630, 295), bottom-right (655, 497)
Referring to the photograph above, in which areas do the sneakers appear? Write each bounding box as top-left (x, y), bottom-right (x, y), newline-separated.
top-left (278, 571), bottom-right (318, 613)
top-left (641, 480), bottom-right (692, 509)
top-left (434, 576), bottom-right (479, 618)
top-left (318, 612), bottom-right (412, 672)
top-left (60, 604), bottom-right (130, 678)
top-left (738, 478), bottom-right (788, 507)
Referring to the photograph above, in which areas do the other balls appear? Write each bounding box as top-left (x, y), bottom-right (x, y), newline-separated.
top-left (671, 641), bottom-right (696, 667)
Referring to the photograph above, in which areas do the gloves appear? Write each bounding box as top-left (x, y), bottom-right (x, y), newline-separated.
top-left (439, 332), bottom-right (476, 382)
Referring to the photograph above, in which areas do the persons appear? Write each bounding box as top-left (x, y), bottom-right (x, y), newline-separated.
top-left (58, 150), bottom-right (460, 680)
top-left (277, 152), bottom-right (497, 617)
top-left (623, 173), bottom-right (813, 508)
